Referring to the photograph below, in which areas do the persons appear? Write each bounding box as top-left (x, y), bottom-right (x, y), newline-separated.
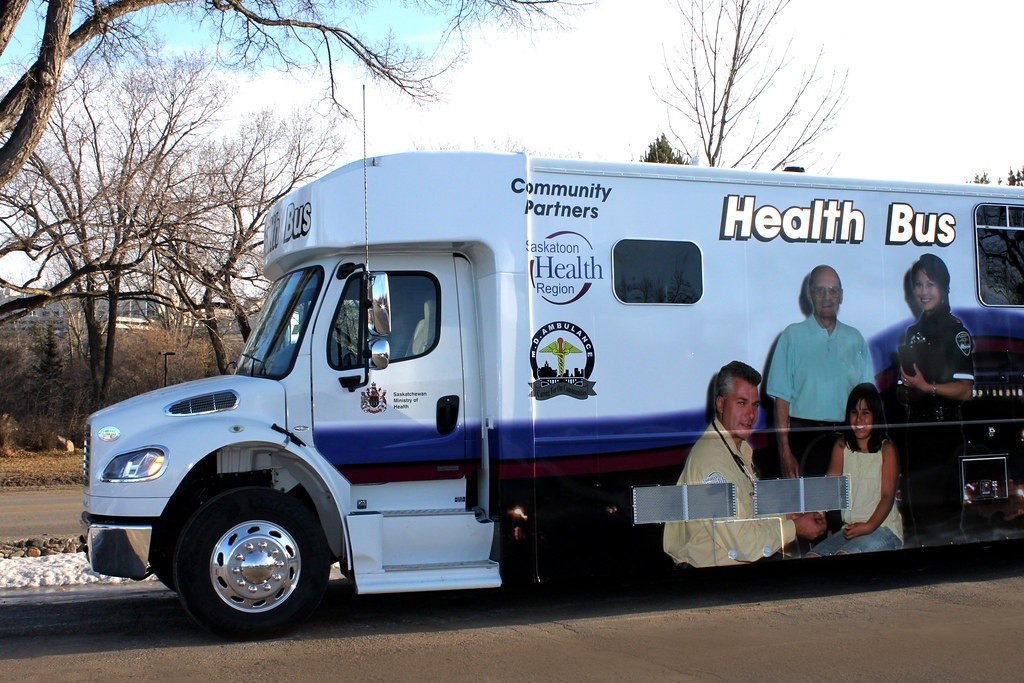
top-left (767, 265), bottom-right (881, 556)
top-left (663, 361), bottom-right (826, 569)
top-left (805, 383), bottom-right (905, 557)
top-left (876, 252), bottom-right (975, 538)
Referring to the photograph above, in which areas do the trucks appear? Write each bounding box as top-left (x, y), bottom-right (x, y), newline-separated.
top-left (75, 149), bottom-right (1023, 641)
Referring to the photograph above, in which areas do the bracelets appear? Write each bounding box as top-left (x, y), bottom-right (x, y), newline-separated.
top-left (931, 385), bottom-right (937, 397)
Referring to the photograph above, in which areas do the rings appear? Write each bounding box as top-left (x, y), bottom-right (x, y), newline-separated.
top-left (904, 379), bottom-right (906, 382)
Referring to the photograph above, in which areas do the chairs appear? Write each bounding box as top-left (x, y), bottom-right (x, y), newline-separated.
top-left (405, 300), bottom-right (436, 357)
